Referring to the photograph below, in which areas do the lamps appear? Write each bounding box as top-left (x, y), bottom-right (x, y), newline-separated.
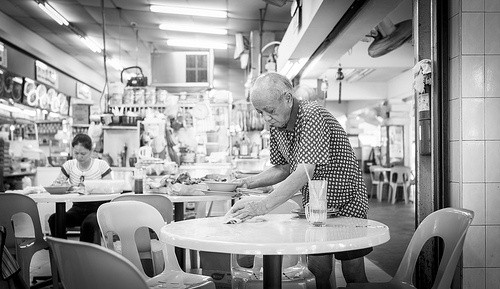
top-left (290, 0), bottom-right (302, 18)
top-left (36, 0), bottom-right (70, 27)
top-left (79, 34), bottom-right (102, 54)
top-left (146, 4), bottom-right (228, 19)
top-left (164, 39), bottom-right (229, 50)
top-left (157, 23), bottom-right (229, 36)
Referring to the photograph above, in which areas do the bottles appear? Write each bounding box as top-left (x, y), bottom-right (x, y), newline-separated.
top-left (37, 123), bottom-right (62, 144)
top-left (232, 137), bottom-right (249, 157)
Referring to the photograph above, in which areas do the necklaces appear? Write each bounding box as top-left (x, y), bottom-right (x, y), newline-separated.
top-left (78, 158), bottom-right (92, 172)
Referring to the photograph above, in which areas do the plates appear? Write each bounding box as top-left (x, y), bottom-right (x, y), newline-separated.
top-left (4, 81), bottom-right (69, 115)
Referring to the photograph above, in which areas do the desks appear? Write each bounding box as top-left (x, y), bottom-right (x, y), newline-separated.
top-left (25, 194), bottom-right (239, 289)
top-left (158, 213), bottom-right (390, 289)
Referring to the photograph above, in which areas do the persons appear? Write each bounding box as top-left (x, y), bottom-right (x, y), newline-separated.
top-left (230, 72), bottom-right (373, 289)
top-left (48, 134), bottom-right (112, 245)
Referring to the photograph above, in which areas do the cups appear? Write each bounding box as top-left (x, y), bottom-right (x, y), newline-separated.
top-left (134, 167), bottom-right (143, 194)
top-left (306, 180), bottom-right (327, 227)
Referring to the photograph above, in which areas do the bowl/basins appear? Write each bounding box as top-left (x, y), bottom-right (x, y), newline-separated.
top-left (206, 182), bottom-right (237, 191)
top-left (44, 185), bottom-right (68, 195)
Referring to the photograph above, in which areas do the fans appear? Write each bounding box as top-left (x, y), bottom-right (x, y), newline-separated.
top-left (366, 15), bottom-right (413, 59)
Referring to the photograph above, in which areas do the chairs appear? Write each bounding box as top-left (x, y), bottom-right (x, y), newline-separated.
top-left (96, 201), bottom-right (216, 289)
top-left (387, 165), bottom-right (415, 205)
top-left (369, 166), bottom-right (397, 204)
top-left (109, 194), bottom-right (173, 277)
top-left (341, 207), bottom-right (475, 289)
top-left (224, 194), bottom-right (314, 289)
top-left (0, 192), bottom-right (60, 289)
top-left (44, 236), bottom-right (150, 289)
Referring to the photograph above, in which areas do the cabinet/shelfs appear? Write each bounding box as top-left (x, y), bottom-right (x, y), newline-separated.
top-left (0, 37), bottom-right (272, 231)
